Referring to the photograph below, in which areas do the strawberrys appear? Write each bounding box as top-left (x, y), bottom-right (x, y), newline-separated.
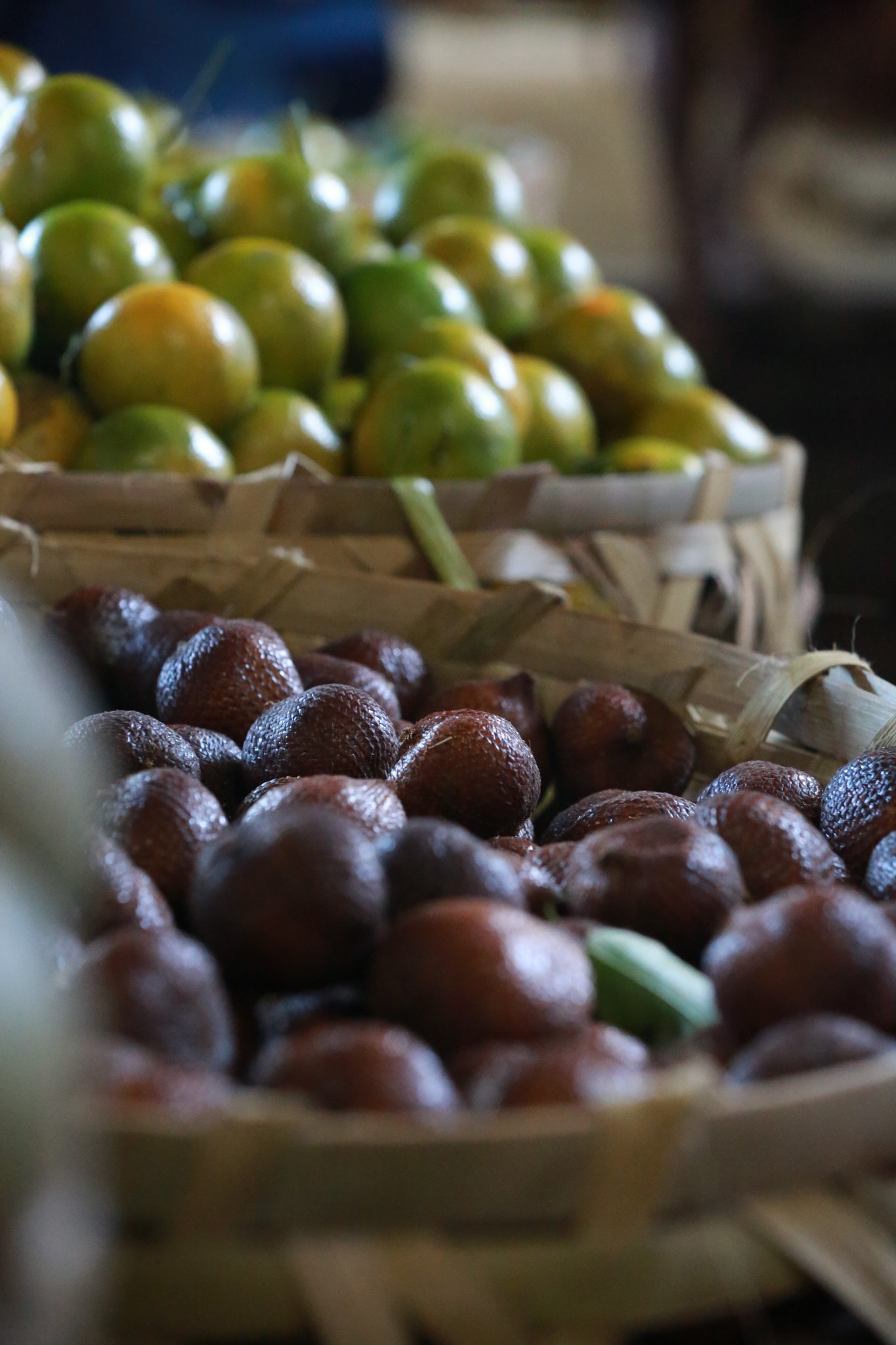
top-left (16, 577), bottom-right (896, 1115)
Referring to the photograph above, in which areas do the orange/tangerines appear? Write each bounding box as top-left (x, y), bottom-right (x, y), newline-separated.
top-left (0, 45), bottom-right (775, 475)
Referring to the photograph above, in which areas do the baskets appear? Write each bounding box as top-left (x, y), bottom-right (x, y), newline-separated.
top-left (0, 441), bottom-right (804, 661)
top-left (0, 528), bottom-right (896, 1342)
